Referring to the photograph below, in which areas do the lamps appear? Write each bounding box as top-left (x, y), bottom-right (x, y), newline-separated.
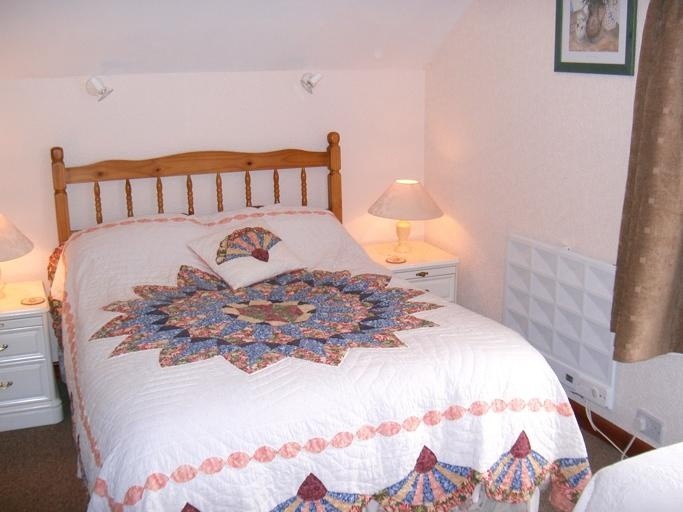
top-left (367, 180), bottom-right (444, 253)
top-left (0, 216), bottom-right (31, 289)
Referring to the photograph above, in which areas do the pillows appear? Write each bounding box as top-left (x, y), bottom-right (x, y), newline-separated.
top-left (187, 220), bottom-right (305, 288)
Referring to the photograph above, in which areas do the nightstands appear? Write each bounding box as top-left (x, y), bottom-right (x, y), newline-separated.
top-left (367, 237), bottom-right (457, 304)
top-left (0, 281), bottom-right (65, 433)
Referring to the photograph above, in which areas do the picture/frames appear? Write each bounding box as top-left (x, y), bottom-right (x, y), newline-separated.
top-left (555, 1), bottom-right (636, 76)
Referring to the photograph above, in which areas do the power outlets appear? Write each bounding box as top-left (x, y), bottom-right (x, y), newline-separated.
top-left (588, 379), bottom-right (610, 408)
top-left (633, 408), bottom-right (663, 443)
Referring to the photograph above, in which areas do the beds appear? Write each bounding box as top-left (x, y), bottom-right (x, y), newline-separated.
top-left (42, 132), bottom-right (589, 512)
